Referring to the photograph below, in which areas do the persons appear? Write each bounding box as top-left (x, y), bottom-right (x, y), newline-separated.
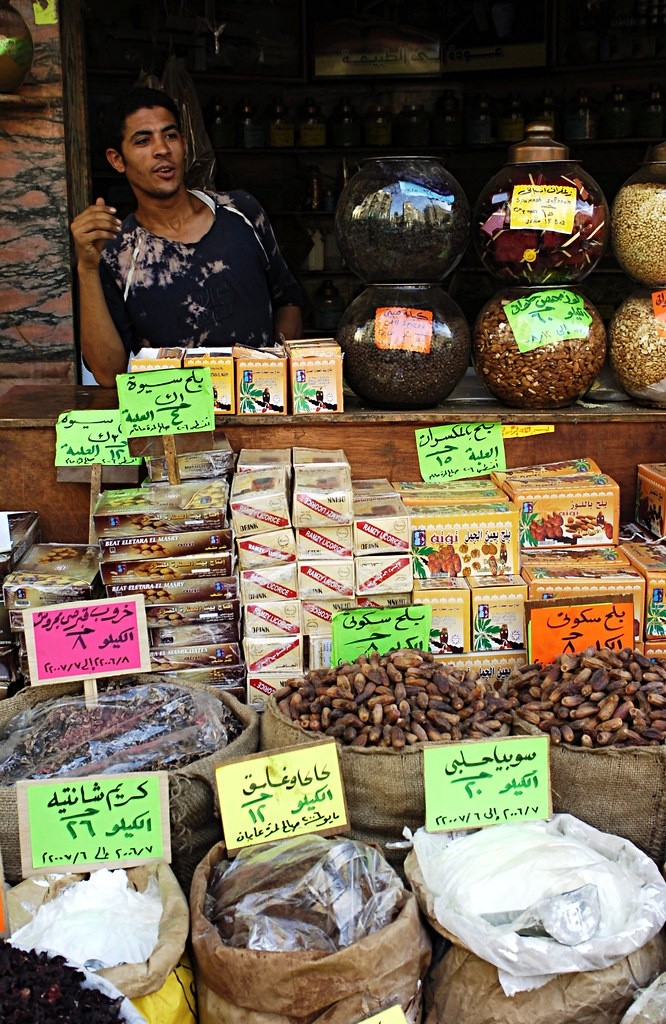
top-left (70, 83), bottom-right (301, 390)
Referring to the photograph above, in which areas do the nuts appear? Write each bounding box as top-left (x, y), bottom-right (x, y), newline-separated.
top-left (470, 294), bottom-right (666, 409)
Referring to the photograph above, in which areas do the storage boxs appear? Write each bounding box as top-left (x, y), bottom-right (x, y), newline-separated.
top-left (0, 338), bottom-right (666, 711)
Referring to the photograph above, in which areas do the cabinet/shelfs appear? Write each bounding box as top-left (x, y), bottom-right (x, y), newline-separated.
top-left (82, 0), bottom-right (666, 339)
